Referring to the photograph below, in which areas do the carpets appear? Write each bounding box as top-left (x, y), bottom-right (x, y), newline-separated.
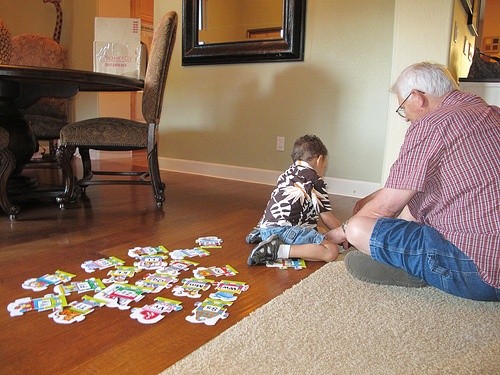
top-left (147, 240), bottom-right (500, 375)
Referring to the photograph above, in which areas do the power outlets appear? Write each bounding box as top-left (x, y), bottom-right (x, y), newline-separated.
top-left (276, 137), bottom-right (285, 150)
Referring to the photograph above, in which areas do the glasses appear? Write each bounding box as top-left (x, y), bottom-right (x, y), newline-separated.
top-left (396, 90), bottom-right (426, 118)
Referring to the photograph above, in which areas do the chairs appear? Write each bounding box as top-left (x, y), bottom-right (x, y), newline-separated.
top-left (0, 0), bottom-right (178, 209)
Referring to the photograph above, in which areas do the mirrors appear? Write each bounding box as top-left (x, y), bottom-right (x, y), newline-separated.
top-left (181, 0), bottom-right (308, 67)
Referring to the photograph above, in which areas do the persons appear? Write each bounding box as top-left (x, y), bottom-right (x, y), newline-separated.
top-left (246, 134), bottom-right (348, 265)
top-left (327, 62), bottom-right (500, 302)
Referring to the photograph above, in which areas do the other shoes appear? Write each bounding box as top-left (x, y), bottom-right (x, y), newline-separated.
top-left (343, 250), bottom-right (429, 288)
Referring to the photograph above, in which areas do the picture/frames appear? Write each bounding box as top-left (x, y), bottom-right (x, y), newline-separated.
top-left (452, 22), bottom-right (460, 42)
top-left (468, 44), bottom-right (473, 62)
top-left (463, 37), bottom-right (468, 55)
top-left (462, 0), bottom-right (482, 36)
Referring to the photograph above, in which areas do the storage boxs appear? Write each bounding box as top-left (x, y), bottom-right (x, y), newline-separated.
top-left (92, 15), bottom-right (144, 80)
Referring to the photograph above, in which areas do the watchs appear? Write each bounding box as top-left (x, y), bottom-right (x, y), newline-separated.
top-left (342, 221), bottom-right (347, 233)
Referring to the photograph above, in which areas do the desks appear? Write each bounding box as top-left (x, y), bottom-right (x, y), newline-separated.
top-left (0, 64), bottom-right (138, 222)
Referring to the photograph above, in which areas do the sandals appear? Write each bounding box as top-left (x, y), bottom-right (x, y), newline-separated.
top-left (246, 229), bottom-right (263, 244)
top-left (247, 234), bottom-right (284, 265)
